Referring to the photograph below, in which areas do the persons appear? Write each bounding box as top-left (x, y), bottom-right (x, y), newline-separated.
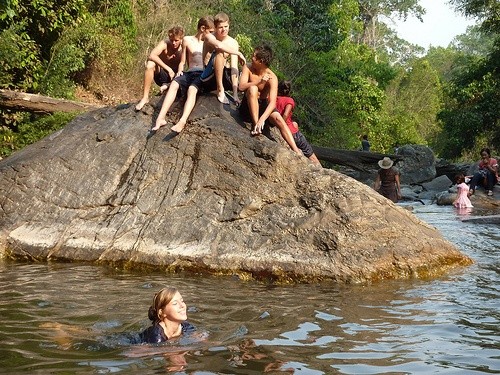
top-left (394, 143), bottom-right (400, 154)
top-left (362, 135), bottom-right (370, 151)
top-left (199, 13), bottom-right (246, 104)
top-left (131, 287), bottom-right (190, 343)
top-left (374, 157), bottom-right (401, 204)
top-left (152, 17), bottom-right (214, 132)
top-left (469, 148), bottom-right (498, 196)
top-left (262, 86), bottom-right (320, 165)
top-left (136, 25), bottom-right (184, 110)
top-left (448, 175), bottom-right (475, 208)
top-left (240, 45), bottom-right (303, 155)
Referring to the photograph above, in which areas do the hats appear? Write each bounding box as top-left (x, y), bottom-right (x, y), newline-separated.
top-left (378, 157), bottom-right (394, 169)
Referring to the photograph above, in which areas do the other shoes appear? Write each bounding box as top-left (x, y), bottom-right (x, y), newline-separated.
top-left (469, 189), bottom-right (474, 195)
top-left (488, 190), bottom-right (493, 195)
